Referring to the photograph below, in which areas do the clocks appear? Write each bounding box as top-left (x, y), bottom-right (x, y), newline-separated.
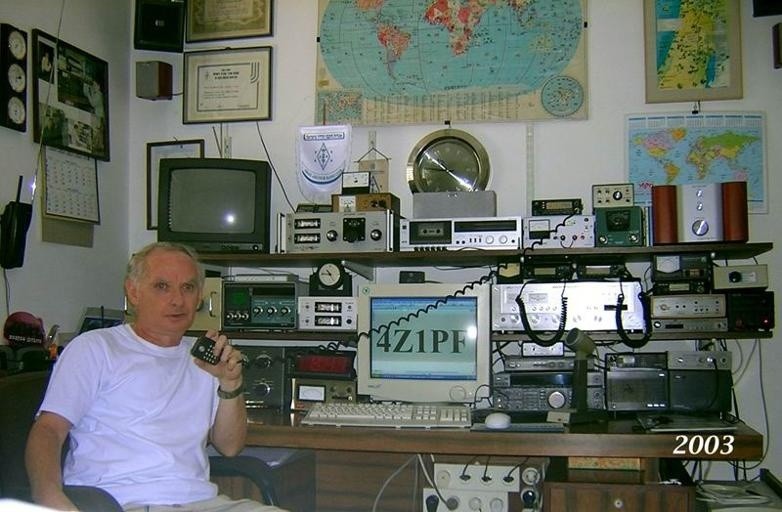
top-left (406, 130), bottom-right (490, 191)
top-left (306, 263), bottom-right (351, 296)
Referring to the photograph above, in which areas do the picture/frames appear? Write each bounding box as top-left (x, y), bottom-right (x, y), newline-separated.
top-left (146, 139), bottom-right (206, 230)
top-left (31, 28), bottom-right (110, 163)
top-left (185, 0), bottom-right (272, 44)
top-left (182, 45), bottom-right (273, 124)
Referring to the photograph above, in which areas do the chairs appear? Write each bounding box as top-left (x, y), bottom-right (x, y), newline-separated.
top-left (0, 366), bottom-right (279, 512)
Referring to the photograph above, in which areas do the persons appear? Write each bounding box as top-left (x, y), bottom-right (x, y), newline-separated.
top-left (42, 51), bottom-right (52, 74)
top-left (90, 79), bottom-right (105, 149)
top-left (24, 243), bottom-right (293, 512)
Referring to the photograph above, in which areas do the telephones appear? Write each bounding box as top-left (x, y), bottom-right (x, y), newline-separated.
top-left (0, 176), bottom-right (33, 269)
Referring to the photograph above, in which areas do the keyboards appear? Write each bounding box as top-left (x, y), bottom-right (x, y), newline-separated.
top-left (302, 400), bottom-right (473, 434)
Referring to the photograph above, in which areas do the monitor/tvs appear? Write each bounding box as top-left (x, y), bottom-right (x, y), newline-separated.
top-left (157, 157), bottom-right (271, 253)
top-left (357, 282), bottom-right (492, 406)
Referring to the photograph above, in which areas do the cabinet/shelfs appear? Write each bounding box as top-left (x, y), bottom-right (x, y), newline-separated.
top-left (190, 243), bottom-right (776, 511)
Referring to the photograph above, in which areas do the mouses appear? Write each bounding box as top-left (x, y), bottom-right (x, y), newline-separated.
top-left (485, 411), bottom-right (511, 430)
